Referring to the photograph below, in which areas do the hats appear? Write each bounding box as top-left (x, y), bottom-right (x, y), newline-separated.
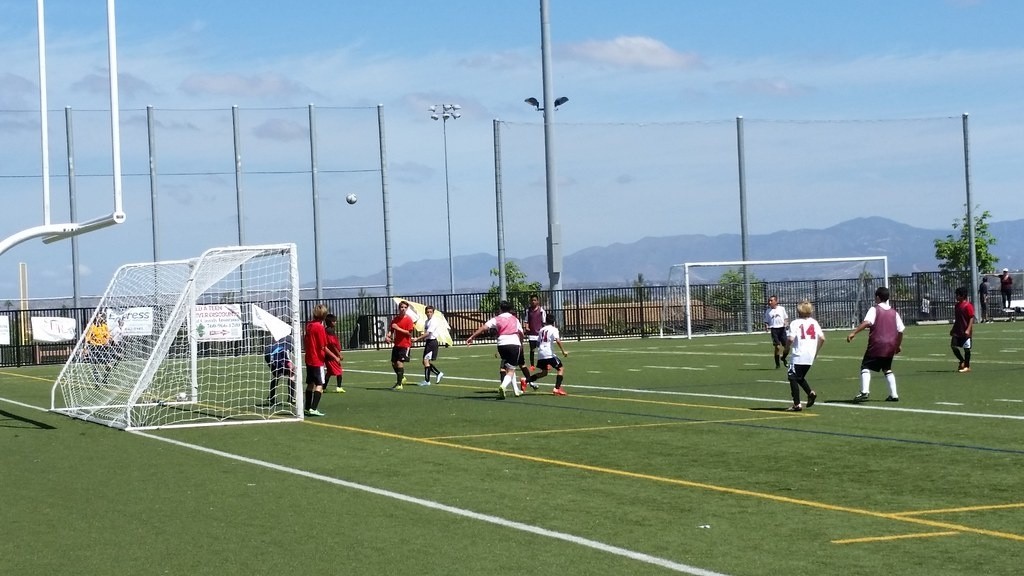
top-left (1003, 268), bottom-right (1008, 273)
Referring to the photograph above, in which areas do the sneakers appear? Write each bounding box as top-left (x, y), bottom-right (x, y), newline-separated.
top-left (499, 384), bottom-right (507, 399)
top-left (418, 380), bottom-right (431, 386)
top-left (520, 376), bottom-right (528, 391)
top-left (513, 390), bottom-right (524, 397)
top-left (552, 387), bottom-right (568, 396)
top-left (530, 383), bottom-right (539, 390)
top-left (436, 372), bottom-right (444, 384)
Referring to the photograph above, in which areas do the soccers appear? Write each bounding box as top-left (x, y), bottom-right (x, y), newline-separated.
top-left (346, 194), bottom-right (357, 204)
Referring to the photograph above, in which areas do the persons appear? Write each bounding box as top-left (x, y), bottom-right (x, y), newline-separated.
top-left (500, 310), bottom-right (539, 390)
top-left (411, 306), bottom-right (444, 386)
top-left (322, 314), bottom-right (346, 393)
top-left (847, 287), bottom-right (905, 402)
top-left (109, 320), bottom-right (125, 371)
top-left (304, 305), bottom-right (341, 417)
top-left (86, 312), bottom-right (110, 390)
top-left (466, 301), bottom-right (524, 400)
top-left (265, 334), bottom-right (296, 406)
top-left (993, 268), bottom-right (1013, 308)
top-left (949, 287), bottom-right (975, 373)
top-left (979, 276), bottom-right (990, 323)
top-left (525, 295), bottom-right (551, 371)
top-left (520, 314), bottom-right (568, 395)
top-left (764, 295), bottom-right (790, 369)
top-left (386, 301), bottom-right (414, 391)
top-left (780, 302), bottom-right (825, 411)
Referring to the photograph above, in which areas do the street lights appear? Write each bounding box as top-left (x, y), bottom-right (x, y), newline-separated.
top-left (525, 96), bottom-right (570, 333)
top-left (427, 103), bottom-right (463, 309)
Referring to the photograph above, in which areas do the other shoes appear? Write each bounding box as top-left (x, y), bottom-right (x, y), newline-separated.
top-left (786, 404), bottom-right (803, 412)
top-left (546, 365), bottom-right (552, 371)
top-left (401, 377), bottom-right (407, 384)
top-left (805, 390), bottom-right (818, 407)
top-left (389, 382), bottom-right (404, 391)
top-left (981, 319), bottom-right (991, 323)
top-left (323, 387), bottom-right (328, 392)
top-left (781, 357), bottom-right (788, 367)
top-left (307, 408), bottom-right (326, 417)
top-left (885, 394), bottom-right (899, 402)
top-left (304, 408), bottom-right (312, 415)
top-left (530, 366), bottom-right (536, 371)
top-left (288, 398), bottom-right (296, 404)
top-left (775, 362), bottom-right (780, 368)
top-left (267, 396), bottom-right (278, 406)
top-left (335, 385), bottom-right (346, 393)
top-left (957, 361), bottom-right (970, 373)
top-left (852, 391), bottom-right (869, 402)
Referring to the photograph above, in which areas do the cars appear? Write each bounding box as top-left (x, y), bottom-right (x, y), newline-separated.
top-left (1004, 294), bottom-right (1024, 317)
